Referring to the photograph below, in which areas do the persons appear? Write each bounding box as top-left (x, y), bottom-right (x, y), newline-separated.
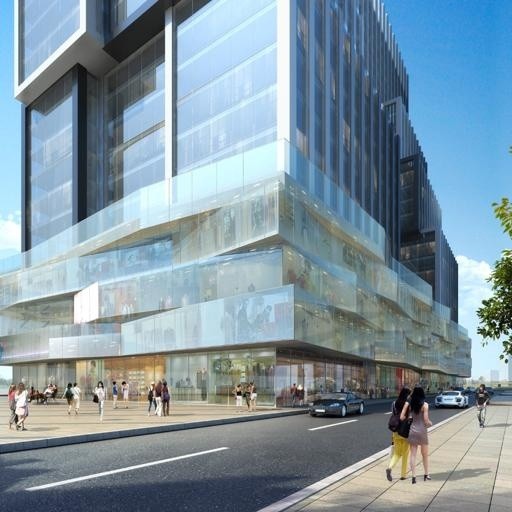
top-left (7, 383), bottom-right (18, 429)
top-left (297, 384), bottom-right (305, 407)
top-left (121, 380), bottom-right (131, 408)
top-left (155, 381), bottom-right (163, 416)
top-left (15, 383), bottom-right (29, 431)
top-left (384, 385), bottom-right (409, 481)
top-left (368, 384), bottom-right (392, 400)
top-left (62, 382), bottom-right (73, 415)
top-left (243, 382), bottom-right (251, 410)
top-left (238, 298), bottom-right (252, 339)
top-left (232, 384), bottom-right (244, 412)
top-left (37, 383), bottom-right (53, 394)
top-left (43, 385), bottom-right (58, 405)
top-left (401, 387), bottom-right (438, 484)
top-left (89, 361), bottom-right (97, 378)
top-left (161, 380), bottom-right (172, 417)
top-left (247, 381), bottom-right (258, 412)
top-left (252, 305), bottom-right (272, 332)
top-left (72, 382), bottom-right (81, 415)
top-left (290, 383), bottom-right (297, 407)
top-left (475, 383), bottom-right (490, 428)
top-left (111, 381), bottom-right (119, 408)
top-left (29, 386), bottom-right (36, 402)
top-left (148, 381), bottom-right (157, 416)
top-left (93, 380), bottom-right (106, 419)
